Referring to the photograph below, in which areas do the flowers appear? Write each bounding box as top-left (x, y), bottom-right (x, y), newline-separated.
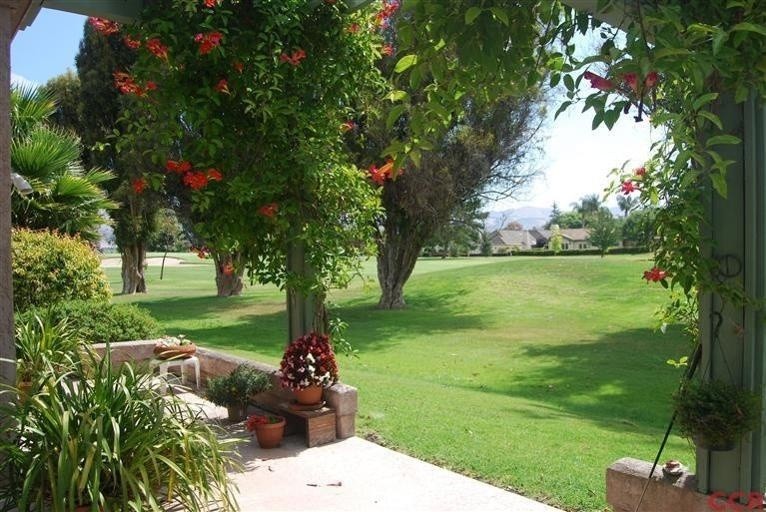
top-left (278, 331), bottom-right (339, 392)
top-left (243, 414), bottom-right (279, 432)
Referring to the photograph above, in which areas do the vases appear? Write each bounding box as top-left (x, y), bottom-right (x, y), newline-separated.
top-left (254, 415), bottom-right (286, 449)
top-left (292, 382), bottom-right (322, 404)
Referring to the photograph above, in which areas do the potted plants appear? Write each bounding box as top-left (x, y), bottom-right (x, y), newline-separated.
top-left (669, 377), bottom-right (764, 453)
top-left (201, 360), bottom-right (276, 422)
